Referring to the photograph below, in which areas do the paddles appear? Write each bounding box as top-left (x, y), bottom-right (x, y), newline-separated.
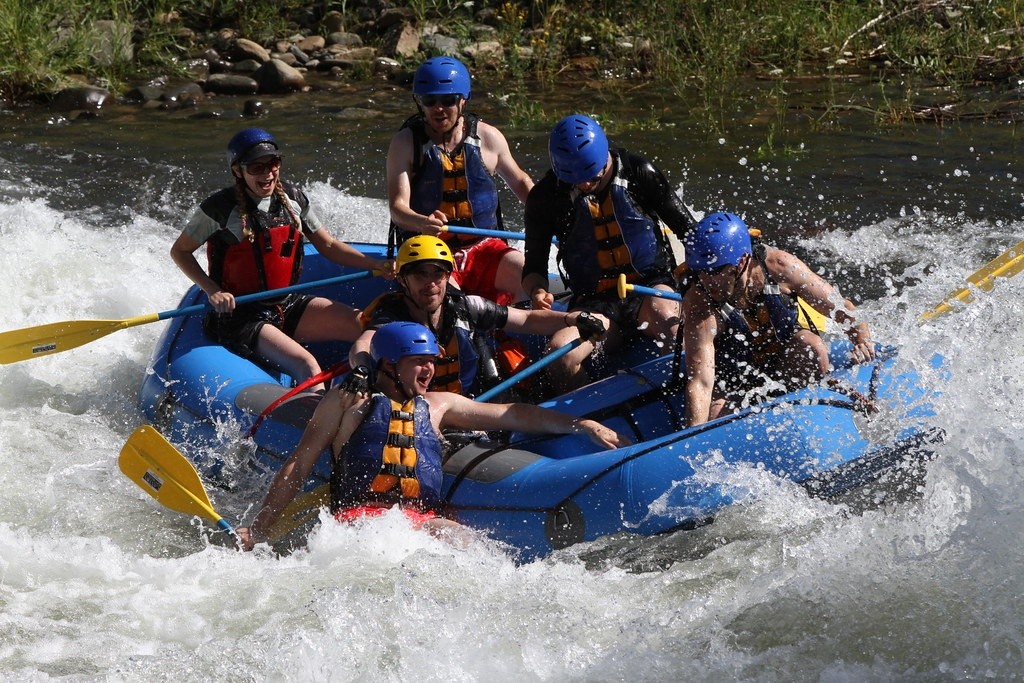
top-left (268, 331), bottom-right (600, 544)
top-left (617, 273), bottom-right (826, 332)
top-left (118, 425), bottom-right (236, 538)
top-left (918, 240), bottom-right (1024, 326)
top-left (442, 224), bottom-right (762, 242)
top-left (0, 262), bottom-right (390, 366)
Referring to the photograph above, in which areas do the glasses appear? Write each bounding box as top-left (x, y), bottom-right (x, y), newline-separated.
top-left (587, 167), bottom-right (607, 184)
top-left (702, 265), bottom-right (730, 276)
top-left (406, 267), bottom-right (448, 282)
top-left (419, 96), bottom-right (460, 107)
top-left (238, 157), bottom-right (282, 175)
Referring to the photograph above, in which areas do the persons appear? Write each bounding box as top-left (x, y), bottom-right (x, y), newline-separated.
top-left (519, 115), bottom-right (696, 355)
top-left (384, 55), bottom-right (536, 310)
top-left (678, 210), bottom-right (877, 433)
top-left (231, 226), bottom-right (612, 556)
top-left (172, 128), bottom-right (401, 396)
top-left (322, 317), bottom-right (638, 539)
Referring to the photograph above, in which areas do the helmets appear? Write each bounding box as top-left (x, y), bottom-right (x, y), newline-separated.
top-left (370, 321), bottom-right (442, 366)
top-left (227, 129), bottom-right (285, 179)
top-left (412, 56), bottom-right (471, 99)
top-left (687, 212), bottom-right (753, 269)
top-left (550, 114), bottom-right (610, 184)
top-left (395, 235), bottom-right (454, 277)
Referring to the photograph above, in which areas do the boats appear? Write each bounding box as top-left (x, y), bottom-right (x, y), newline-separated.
top-left (142, 238), bottom-right (954, 559)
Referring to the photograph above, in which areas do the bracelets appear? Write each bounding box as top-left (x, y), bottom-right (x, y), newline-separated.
top-left (564, 312), bottom-right (572, 327)
